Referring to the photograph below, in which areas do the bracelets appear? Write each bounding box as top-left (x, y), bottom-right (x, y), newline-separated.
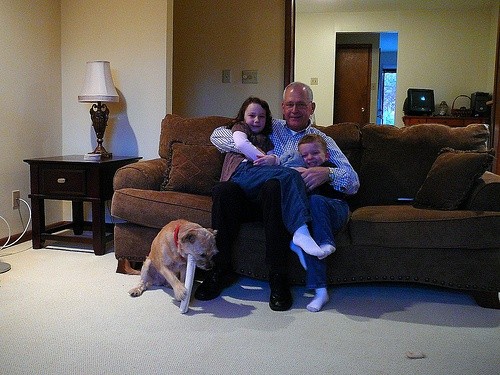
top-left (327, 167), bottom-right (334, 181)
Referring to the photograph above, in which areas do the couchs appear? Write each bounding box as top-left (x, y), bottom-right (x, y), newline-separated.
top-left (109, 112), bottom-right (500, 309)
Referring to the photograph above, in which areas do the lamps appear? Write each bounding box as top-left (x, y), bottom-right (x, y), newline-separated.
top-left (78, 60), bottom-right (120, 157)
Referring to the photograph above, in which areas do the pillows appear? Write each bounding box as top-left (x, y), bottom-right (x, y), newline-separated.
top-left (412, 146), bottom-right (496, 210)
top-left (160, 140), bottom-right (224, 194)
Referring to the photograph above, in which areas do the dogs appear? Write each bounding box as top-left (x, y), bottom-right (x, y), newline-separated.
top-left (126, 217), bottom-right (220, 303)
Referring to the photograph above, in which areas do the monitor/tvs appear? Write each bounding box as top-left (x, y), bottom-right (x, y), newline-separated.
top-left (407, 88), bottom-right (435, 116)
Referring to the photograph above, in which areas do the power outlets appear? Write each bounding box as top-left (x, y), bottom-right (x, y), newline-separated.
top-left (12, 190), bottom-right (20, 209)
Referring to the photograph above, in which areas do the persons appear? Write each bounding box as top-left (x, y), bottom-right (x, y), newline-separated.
top-left (193, 82), bottom-right (361, 311)
top-left (287, 133), bottom-right (350, 259)
top-left (218, 96), bottom-right (323, 270)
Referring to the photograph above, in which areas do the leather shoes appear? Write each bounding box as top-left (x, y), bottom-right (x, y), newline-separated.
top-left (194, 271), bottom-right (235, 300)
top-left (269, 273), bottom-right (293, 311)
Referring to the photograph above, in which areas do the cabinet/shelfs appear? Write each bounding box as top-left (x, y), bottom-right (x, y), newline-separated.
top-left (402, 116), bottom-right (487, 127)
top-left (25, 155), bottom-right (142, 255)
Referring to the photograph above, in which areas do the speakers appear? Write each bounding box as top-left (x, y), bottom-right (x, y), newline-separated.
top-left (471, 93), bottom-right (492, 119)
